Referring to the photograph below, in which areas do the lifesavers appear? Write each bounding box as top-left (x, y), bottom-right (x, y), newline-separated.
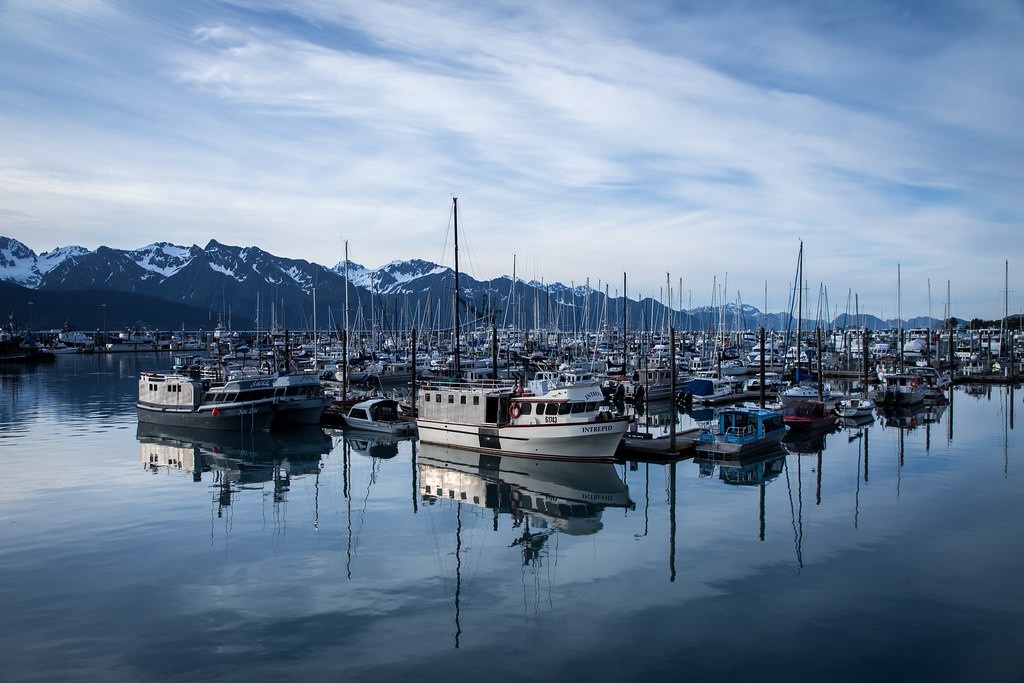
top-left (508, 404), bottom-right (522, 419)
top-left (909, 380), bottom-right (918, 388)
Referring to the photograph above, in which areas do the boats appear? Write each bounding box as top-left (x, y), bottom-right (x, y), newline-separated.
top-left (416, 442), bottom-right (638, 651)
top-left (134, 420), bottom-right (334, 543)
top-left (777, 424), bottom-right (838, 506)
top-left (1, 192), bottom-right (1024, 457)
top-left (689, 443), bottom-right (805, 570)
